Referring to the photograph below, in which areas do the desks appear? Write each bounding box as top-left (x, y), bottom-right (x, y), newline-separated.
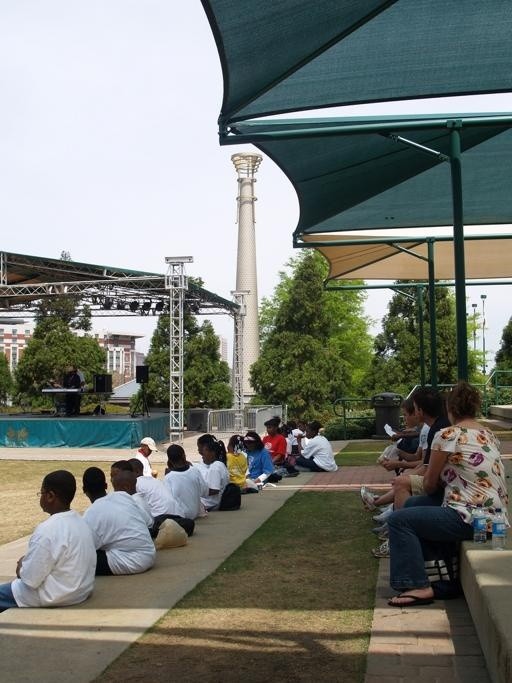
top-left (77, 392), bottom-right (115, 416)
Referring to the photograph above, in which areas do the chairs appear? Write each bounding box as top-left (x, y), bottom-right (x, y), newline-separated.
top-left (53, 392), bottom-right (68, 417)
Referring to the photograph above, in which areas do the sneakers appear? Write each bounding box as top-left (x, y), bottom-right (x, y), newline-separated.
top-left (372, 509), bottom-right (393, 522)
top-left (372, 523), bottom-right (389, 534)
top-left (371, 540), bottom-right (391, 558)
top-left (379, 504), bottom-right (392, 512)
top-left (360, 486), bottom-right (378, 511)
top-left (378, 530), bottom-right (390, 540)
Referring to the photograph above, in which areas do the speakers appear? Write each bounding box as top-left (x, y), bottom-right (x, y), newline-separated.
top-left (136, 366), bottom-right (148, 383)
top-left (96, 374), bottom-right (111, 392)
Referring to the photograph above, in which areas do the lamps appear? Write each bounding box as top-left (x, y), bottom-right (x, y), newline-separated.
top-left (91, 293), bottom-right (200, 316)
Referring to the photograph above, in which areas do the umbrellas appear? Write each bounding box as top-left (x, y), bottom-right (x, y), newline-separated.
top-left (201, 0), bottom-right (512, 124)
top-left (235, 114), bottom-right (512, 382)
top-left (293, 228), bottom-right (512, 387)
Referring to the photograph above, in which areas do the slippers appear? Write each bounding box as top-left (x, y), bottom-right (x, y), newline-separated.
top-left (387, 587), bottom-right (436, 606)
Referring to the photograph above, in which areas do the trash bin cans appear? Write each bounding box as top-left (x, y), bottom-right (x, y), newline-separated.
top-left (371, 392), bottom-right (404, 440)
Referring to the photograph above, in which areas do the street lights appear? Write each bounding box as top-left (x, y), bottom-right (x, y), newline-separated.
top-left (471, 303), bottom-right (478, 349)
top-left (480, 294), bottom-right (487, 374)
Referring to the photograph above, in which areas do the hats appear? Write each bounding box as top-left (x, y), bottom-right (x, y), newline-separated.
top-left (140, 436), bottom-right (158, 452)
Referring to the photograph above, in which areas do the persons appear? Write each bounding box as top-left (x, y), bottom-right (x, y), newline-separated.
top-left (110, 411), bottom-right (341, 539)
top-left (356, 379), bottom-right (453, 557)
top-left (0, 468), bottom-right (96, 608)
top-left (79, 465), bottom-right (157, 578)
top-left (387, 379), bottom-right (510, 608)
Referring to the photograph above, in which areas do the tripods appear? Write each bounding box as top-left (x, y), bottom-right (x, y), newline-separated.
top-left (132, 385), bottom-right (149, 416)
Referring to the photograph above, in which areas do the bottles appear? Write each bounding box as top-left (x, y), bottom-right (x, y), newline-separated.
top-left (470, 503), bottom-right (488, 547)
top-left (378, 446), bottom-right (399, 462)
top-left (490, 507), bottom-right (507, 552)
